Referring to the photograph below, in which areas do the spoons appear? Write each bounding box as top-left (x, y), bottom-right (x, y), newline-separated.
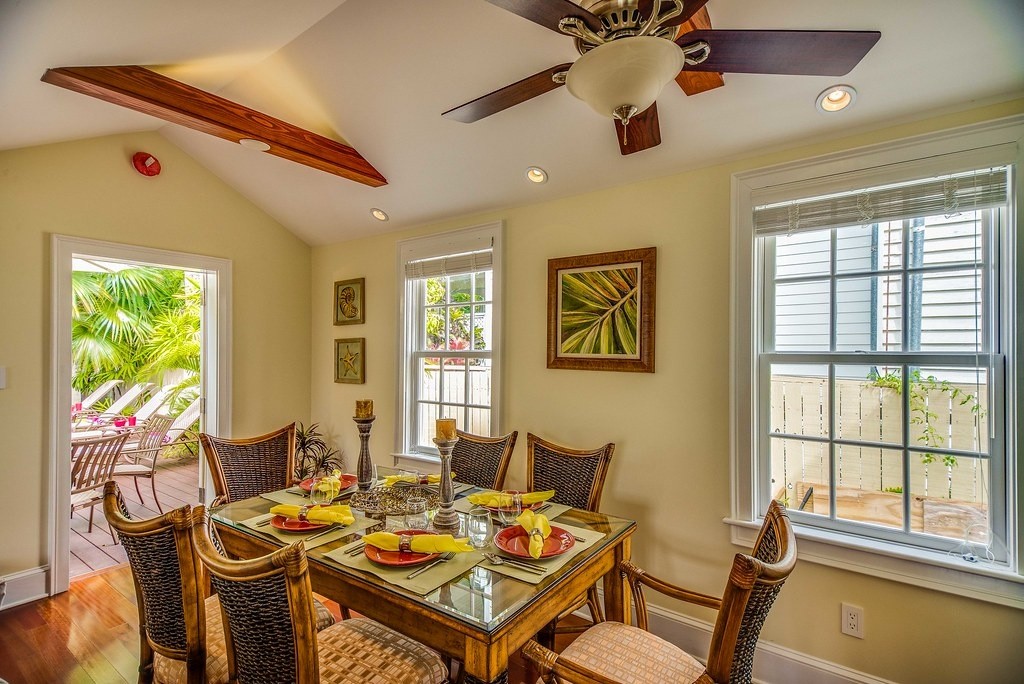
top-left (484, 552), bottom-right (544, 575)
top-left (305, 523), bottom-right (346, 542)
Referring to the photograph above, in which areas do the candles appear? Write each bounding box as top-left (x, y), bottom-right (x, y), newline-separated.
top-left (356, 399), bottom-right (373, 418)
top-left (75, 403), bottom-right (82, 411)
top-left (435, 416), bottom-right (457, 440)
top-left (128, 416), bottom-right (136, 427)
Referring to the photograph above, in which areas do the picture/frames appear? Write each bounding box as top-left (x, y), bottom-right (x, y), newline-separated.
top-left (547, 247), bottom-right (657, 374)
top-left (334, 338), bottom-right (365, 384)
top-left (333, 278), bottom-right (366, 326)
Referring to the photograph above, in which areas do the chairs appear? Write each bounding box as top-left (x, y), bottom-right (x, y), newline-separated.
top-left (70, 379), bottom-right (797, 684)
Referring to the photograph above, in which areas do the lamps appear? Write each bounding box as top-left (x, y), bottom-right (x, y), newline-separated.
top-left (565, 35), bottom-right (685, 145)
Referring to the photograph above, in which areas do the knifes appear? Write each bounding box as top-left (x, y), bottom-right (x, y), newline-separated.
top-left (481, 553), bottom-right (548, 572)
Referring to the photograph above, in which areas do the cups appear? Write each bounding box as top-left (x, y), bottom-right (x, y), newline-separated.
top-left (468, 508), bottom-right (494, 551)
top-left (498, 490), bottom-right (522, 527)
top-left (370, 464), bottom-right (378, 488)
top-left (310, 476), bottom-right (334, 505)
top-left (399, 469), bottom-right (420, 485)
top-left (404, 497), bottom-right (429, 530)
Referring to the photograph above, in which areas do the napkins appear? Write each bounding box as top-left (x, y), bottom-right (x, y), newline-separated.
top-left (380, 472), bottom-right (457, 486)
top-left (516, 509), bottom-right (552, 559)
top-left (361, 532), bottom-right (476, 553)
top-left (270, 504), bottom-right (355, 525)
top-left (318, 469), bottom-right (342, 501)
top-left (465, 489), bottom-right (555, 507)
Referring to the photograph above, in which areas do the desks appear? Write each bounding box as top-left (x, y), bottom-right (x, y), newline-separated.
top-left (208, 466), bottom-right (636, 684)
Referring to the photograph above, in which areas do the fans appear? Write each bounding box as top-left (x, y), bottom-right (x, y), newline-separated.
top-left (440, 0), bottom-right (882, 124)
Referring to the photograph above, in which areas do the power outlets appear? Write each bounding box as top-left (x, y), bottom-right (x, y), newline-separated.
top-left (840, 603), bottom-right (864, 639)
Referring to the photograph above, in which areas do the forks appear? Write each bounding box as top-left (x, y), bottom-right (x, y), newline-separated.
top-left (407, 552), bottom-right (456, 580)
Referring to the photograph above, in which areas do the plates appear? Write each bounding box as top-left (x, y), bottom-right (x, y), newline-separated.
top-left (481, 490), bottom-right (543, 513)
top-left (270, 504), bottom-right (331, 534)
top-left (299, 474), bottom-right (357, 493)
top-left (364, 530), bottom-right (443, 568)
top-left (494, 525), bottom-right (576, 558)
